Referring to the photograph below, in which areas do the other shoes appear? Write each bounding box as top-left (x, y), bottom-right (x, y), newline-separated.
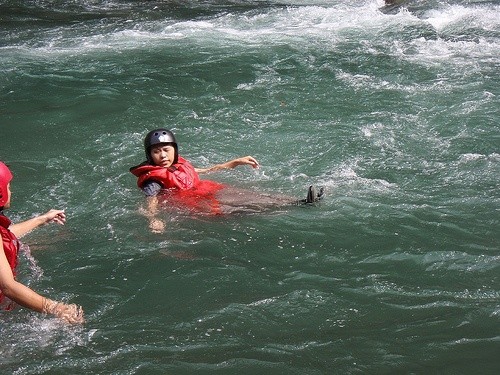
top-left (318, 187), bottom-right (325, 200)
top-left (295, 186), bottom-right (314, 207)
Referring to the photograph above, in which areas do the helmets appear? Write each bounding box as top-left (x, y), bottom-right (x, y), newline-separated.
top-left (0, 161), bottom-right (14, 207)
top-left (144, 129), bottom-right (178, 164)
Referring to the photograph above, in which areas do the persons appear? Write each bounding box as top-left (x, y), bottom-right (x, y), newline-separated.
top-left (0, 160), bottom-right (83, 323)
top-left (129, 128), bottom-right (325, 232)
